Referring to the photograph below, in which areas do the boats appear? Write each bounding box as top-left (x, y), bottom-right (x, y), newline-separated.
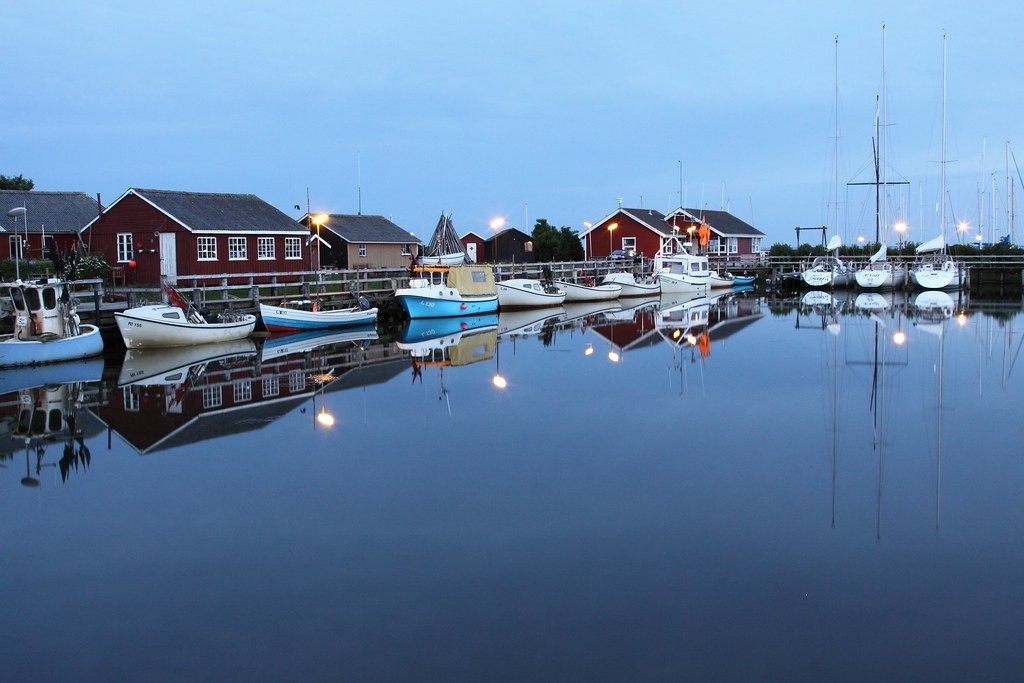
top-left (0, 356), bottom-right (104, 396)
top-left (733, 284), bottom-right (754, 293)
top-left (113, 273), bottom-right (256, 349)
top-left (602, 271), bottom-right (660, 296)
top-left (603, 293), bottom-right (662, 320)
top-left (497, 305), bottom-right (565, 335)
top-left (710, 287), bottom-right (733, 305)
top-left (709, 270), bottom-right (735, 287)
top-left (117, 339), bottom-right (257, 388)
top-left (418, 251), bottom-right (465, 266)
top-left (0, 274), bottom-right (104, 367)
top-left (495, 279), bottom-right (567, 305)
top-left (555, 301), bottom-right (622, 326)
top-left (260, 291), bottom-right (378, 330)
top-left (555, 278), bottom-right (622, 300)
top-left (734, 276), bottom-right (755, 284)
top-left (654, 214), bottom-right (711, 292)
top-left (261, 330), bottom-right (379, 362)
top-left (395, 266), bottom-right (499, 318)
top-left (394, 313), bottom-right (498, 357)
top-left (654, 292), bottom-right (711, 348)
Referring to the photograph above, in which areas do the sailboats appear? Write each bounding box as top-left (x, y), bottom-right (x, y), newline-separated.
top-left (855, 25), bottom-right (908, 288)
top-left (796, 291), bottom-right (957, 543)
top-left (801, 35), bottom-right (854, 286)
top-left (909, 35), bottom-right (966, 289)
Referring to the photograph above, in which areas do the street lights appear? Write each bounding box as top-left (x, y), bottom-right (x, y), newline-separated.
top-left (313, 214), bottom-right (329, 270)
top-left (492, 218), bottom-right (504, 264)
top-left (7, 207), bottom-right (28, 279)
